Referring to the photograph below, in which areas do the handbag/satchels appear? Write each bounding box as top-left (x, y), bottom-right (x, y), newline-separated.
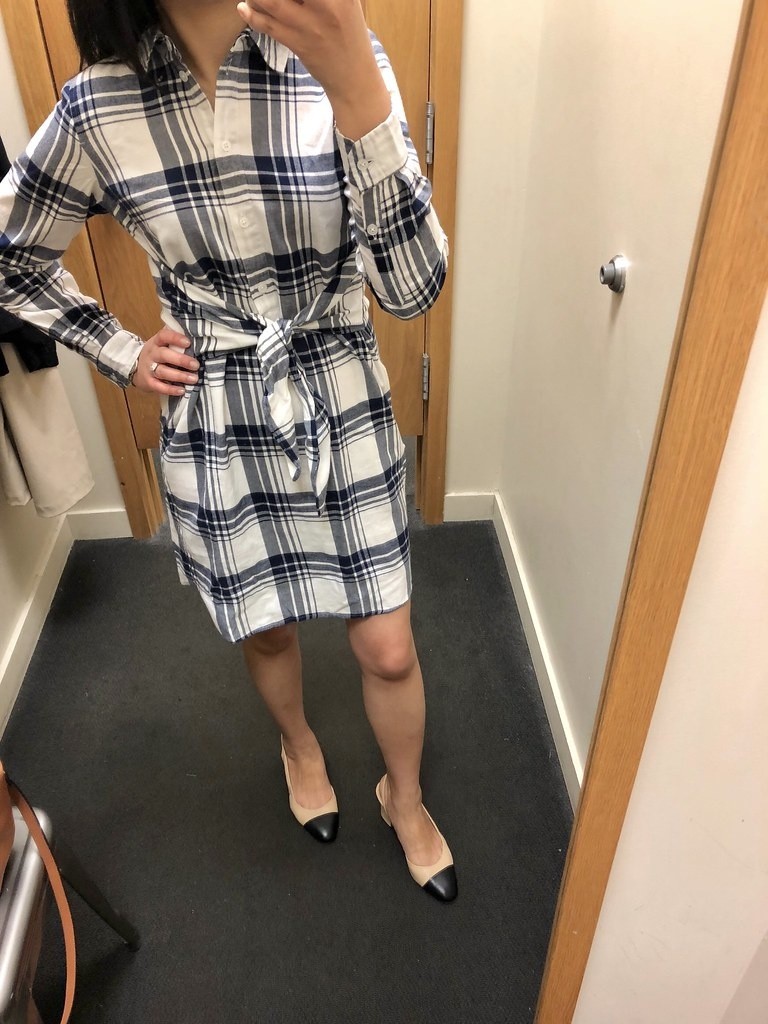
top-left (0, 760), bottom-right (16, 893)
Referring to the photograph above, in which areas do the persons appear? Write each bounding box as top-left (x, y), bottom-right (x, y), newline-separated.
top-left (0, 0), bottom-right (458, 903)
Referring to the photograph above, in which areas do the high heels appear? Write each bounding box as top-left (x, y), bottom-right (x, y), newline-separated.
top-left (374, 773), bottom-right (460, 902)
top-left (279, 732), bottom-right (341, 845)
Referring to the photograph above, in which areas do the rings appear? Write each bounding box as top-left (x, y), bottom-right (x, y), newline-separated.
top-left (149, 363), bottom-right (160, 379)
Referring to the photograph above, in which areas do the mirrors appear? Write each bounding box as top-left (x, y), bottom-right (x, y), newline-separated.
top-left (0, 0), bottom-right (768, 1024)
